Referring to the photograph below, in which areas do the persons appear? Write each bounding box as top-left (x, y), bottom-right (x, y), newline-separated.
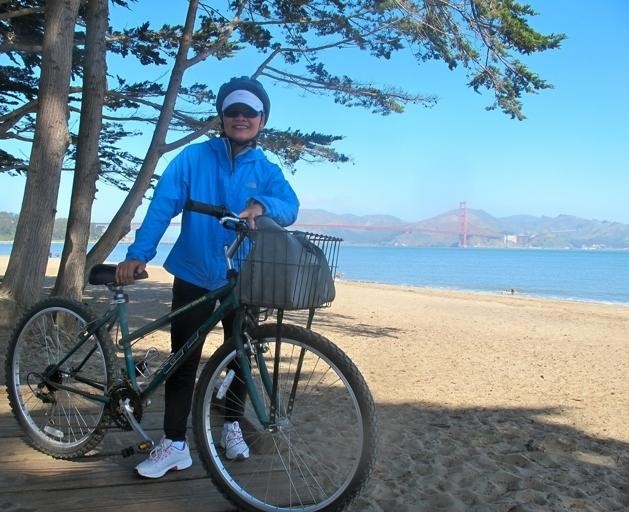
top-left (114, 75), bottom-right (300, 479)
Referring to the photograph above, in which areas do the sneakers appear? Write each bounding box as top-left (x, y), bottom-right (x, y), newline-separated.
top-left (133, 435), bottom-right (193, 479)
top-left (220, 421), bottom-right (250, 460)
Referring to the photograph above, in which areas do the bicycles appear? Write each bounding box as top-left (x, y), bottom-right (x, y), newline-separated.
top-left (4, 199), bottom-right (381, 512)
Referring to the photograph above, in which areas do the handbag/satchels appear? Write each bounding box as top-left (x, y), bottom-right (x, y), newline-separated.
top-left (236, 215), bottom-right (336, 310)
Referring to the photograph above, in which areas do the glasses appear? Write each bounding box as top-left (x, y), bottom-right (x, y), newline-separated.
top-left (224, 107), bottom-right (259, 118)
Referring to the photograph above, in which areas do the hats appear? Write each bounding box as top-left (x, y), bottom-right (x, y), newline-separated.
top-left (216, 75), bottom-right (270, 127)
